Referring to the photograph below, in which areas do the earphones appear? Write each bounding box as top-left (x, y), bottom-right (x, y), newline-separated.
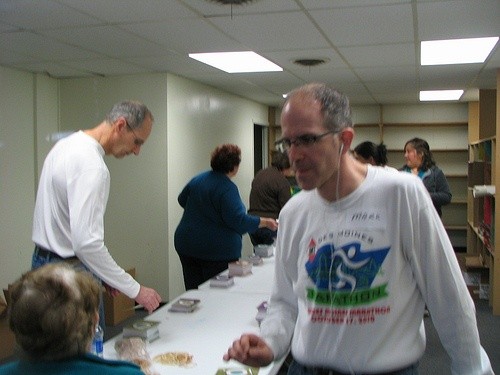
top-left (339, 141), bottom-right (344, 151)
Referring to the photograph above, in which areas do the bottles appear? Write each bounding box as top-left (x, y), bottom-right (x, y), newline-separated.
top-left (88, 326), bottom-right (103, 359)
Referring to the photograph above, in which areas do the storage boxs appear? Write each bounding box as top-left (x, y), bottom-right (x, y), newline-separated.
top-left (1, 284), bottom-right (19, 360)
top-left (101, 268), bottom-right (136, 326)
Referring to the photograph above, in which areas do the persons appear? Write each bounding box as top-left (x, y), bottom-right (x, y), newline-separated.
top-left (0, 262), bottom-right (144, 375)
top-left (32, 100), bottom-right (161, 341)
top-left (247, 148), bottom-right (293, 253)
top-left (399, 137), bottom-right (452, 315)
top-left (353, 138), bottom-right (387, 167)
top-left (223, 84), bottom-right (495, 375)
top-left (174, 144), bottom-right (277, 291)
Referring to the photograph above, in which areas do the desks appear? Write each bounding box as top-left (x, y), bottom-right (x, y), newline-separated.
top-left (99, 244), bottom-right (291, 375)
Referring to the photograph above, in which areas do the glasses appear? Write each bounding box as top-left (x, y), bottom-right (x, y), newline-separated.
top-left (274, 131), bottom-right (334, 147)
top-left (124, 121), bottom-right (146, 145)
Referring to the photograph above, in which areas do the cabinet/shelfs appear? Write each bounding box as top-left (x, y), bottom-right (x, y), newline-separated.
top-left (268, 103), bottom-right (468, 253)
top-left (466, 67), bottom-right (500, 316)
